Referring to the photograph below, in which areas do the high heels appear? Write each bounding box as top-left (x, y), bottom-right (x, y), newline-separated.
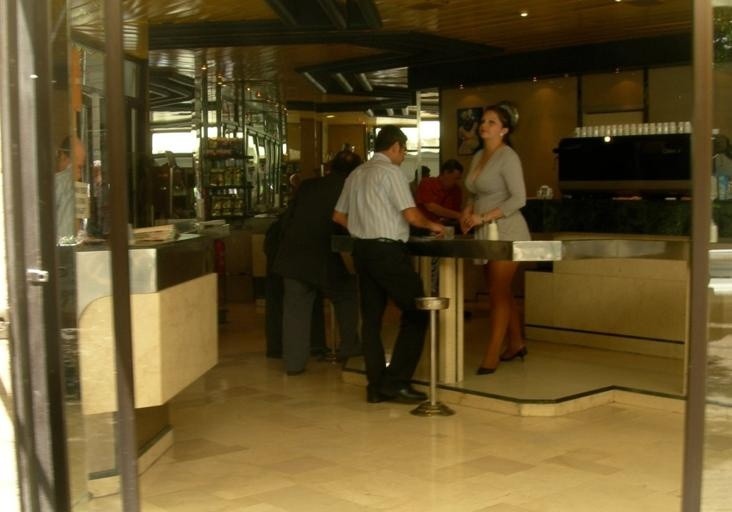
top-left (477, 368), bottom-right (495, 375)
top-left (500, 347), bottom-right (527, 361)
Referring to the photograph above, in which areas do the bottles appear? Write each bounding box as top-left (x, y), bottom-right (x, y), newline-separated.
top-left (574, 120), bottom-right (691, 138)
top-left (488, 218), bottom-right (499, 240)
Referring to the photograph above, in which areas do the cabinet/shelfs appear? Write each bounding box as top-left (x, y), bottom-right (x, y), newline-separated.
top-left (192, 69), bottom-right (288, 223)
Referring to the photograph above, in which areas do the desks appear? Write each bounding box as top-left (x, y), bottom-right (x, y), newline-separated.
top-left (322, 238), bottom-right (564, 387)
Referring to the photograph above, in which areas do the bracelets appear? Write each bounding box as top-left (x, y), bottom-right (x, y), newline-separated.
top-left (481, 213), bottom-right (484, 224)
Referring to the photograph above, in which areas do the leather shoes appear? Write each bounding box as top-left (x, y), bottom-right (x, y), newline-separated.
top-left (336, 346), bottom-right (362, 362)
top-left (381, 385), bottom-right (428, 400)
top-left (367, 393), bottom-right (396, 402)
top-left (267, 347), bottom-right (331, 376)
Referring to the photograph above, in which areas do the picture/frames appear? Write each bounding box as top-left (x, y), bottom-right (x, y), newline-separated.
top-left (456, 106), bottom-right (485, 157)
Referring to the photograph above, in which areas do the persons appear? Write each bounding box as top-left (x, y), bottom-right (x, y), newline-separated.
top-left (260, 160), bottom-right (336, 361)
top-left (459, 104), bottom-right (533, 376)
top-left (258, 148), bottom-right (364, 376)
top-left (53, 136), bottom-right (76, 245)
top-left (409, 163), bottom-right (431, 198)
top-left (412, 159), bottom-right (474, 237)
top-left (330, 122), bottom-right (449, 405)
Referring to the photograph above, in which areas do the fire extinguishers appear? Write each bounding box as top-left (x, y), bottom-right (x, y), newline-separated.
top-left (214, 239), bottom-right (226, 275)
